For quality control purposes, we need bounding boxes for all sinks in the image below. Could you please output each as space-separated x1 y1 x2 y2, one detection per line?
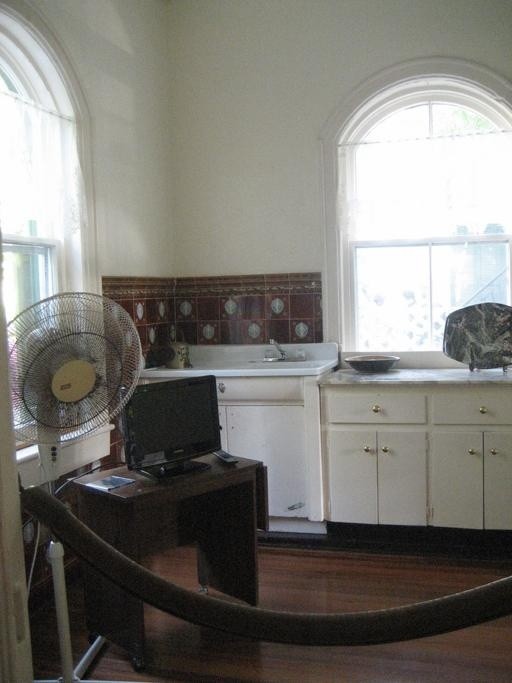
132 361 326 402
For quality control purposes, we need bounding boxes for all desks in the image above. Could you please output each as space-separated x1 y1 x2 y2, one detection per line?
64 452 269 667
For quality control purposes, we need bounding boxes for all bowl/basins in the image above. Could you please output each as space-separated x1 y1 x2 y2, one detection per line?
344 353 404 374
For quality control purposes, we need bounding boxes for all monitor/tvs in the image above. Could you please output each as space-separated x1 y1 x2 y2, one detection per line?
120 375 222 483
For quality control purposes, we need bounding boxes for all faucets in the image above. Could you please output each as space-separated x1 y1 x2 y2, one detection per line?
261 338 289 361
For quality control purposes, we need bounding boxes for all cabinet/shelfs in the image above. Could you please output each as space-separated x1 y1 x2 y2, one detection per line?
316 368 426 542
132 370 336 536
422 367 512 540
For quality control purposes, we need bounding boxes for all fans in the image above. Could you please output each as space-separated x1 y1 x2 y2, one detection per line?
7 292 145 683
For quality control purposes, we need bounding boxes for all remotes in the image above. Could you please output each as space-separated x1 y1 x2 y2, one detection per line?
213 450 239 466
